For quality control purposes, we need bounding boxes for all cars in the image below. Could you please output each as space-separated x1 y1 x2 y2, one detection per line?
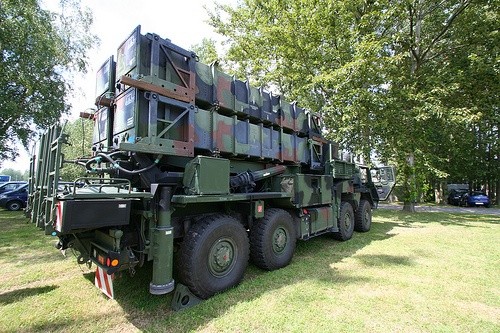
1 182 28 211
446 189 470 206
461 191 491 209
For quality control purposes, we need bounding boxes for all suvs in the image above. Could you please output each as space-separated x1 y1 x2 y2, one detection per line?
0 175 12 185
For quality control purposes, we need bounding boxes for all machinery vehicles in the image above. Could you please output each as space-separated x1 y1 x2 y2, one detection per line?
25 24 397 308
